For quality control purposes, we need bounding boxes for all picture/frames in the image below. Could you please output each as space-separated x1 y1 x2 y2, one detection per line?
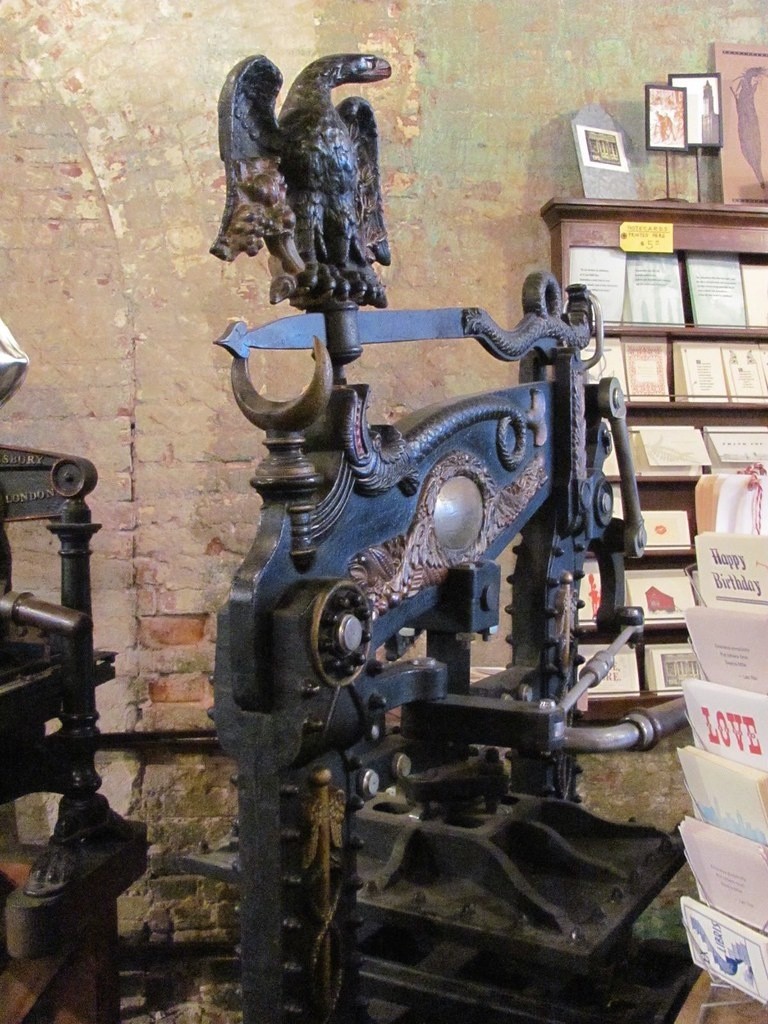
645 84 689 152
667 72 724 148
570 103 637 201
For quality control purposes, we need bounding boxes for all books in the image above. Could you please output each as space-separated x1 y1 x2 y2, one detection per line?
576 555 696 623
693 530 768 606
680 895 767 1005
677 745 768 847
609 476 768 550
579 335 768 405
681 676 767 774
600 416 768 476
684 605 767 696
573 642 710 698
566 244 767 331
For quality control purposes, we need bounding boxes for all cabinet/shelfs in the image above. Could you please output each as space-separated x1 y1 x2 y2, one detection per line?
540 197 768 728
0 445 150 1024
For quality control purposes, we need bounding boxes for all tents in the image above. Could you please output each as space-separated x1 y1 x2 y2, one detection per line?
678 816 768 936
